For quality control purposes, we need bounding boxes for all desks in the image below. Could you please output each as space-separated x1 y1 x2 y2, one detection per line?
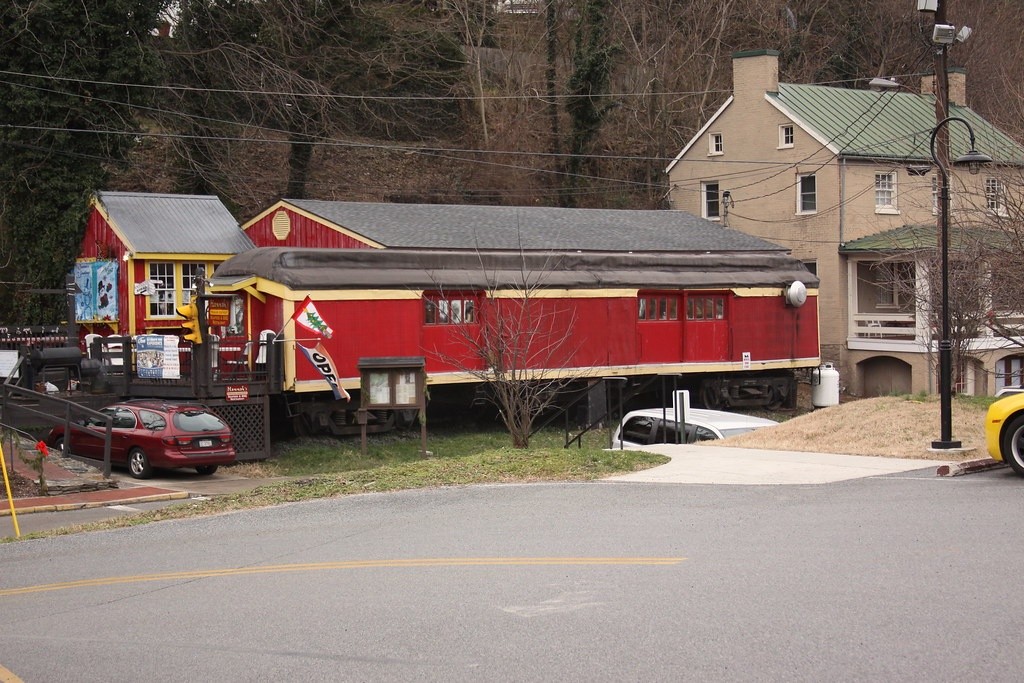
178 346 241 379
882 321 916 338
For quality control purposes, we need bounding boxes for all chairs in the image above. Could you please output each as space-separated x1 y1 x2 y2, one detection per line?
85 332 157 374
864 312 882 339
226 342 254 379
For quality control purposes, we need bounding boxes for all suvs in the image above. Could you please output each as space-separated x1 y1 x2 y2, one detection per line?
48 400 236 479
612 409 778 447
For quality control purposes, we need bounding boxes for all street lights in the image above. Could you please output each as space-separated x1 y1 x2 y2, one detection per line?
930 117 992 450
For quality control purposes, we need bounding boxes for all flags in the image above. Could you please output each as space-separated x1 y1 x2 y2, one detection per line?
297 343 352 404
291 296 333 339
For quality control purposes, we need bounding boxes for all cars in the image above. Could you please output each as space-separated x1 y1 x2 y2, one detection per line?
986 387 1024 476
